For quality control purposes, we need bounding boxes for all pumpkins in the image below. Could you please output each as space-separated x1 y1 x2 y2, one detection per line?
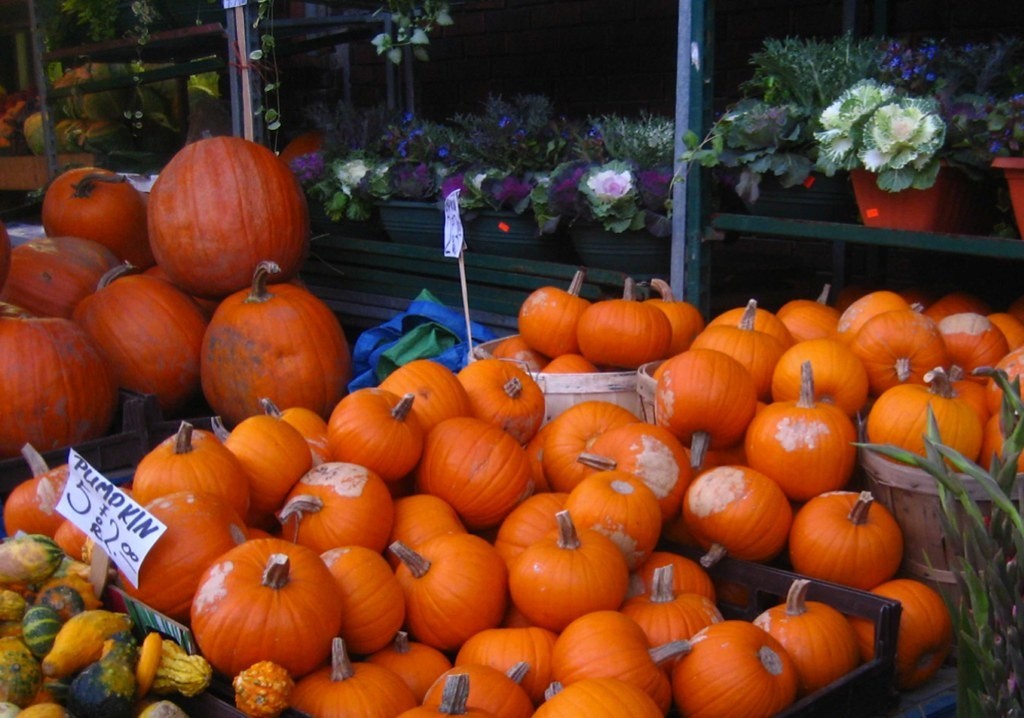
0 138 1024 718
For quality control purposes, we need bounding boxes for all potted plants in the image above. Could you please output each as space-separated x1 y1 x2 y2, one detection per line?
813 78 986 236
983 89 1024 241
664 97 861 223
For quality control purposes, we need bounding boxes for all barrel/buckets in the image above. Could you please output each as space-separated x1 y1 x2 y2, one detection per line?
467 332 638 425
857 413 1024 640
635 358 663 428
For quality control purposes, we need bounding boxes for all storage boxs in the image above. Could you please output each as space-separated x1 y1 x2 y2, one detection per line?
0 335 1024 718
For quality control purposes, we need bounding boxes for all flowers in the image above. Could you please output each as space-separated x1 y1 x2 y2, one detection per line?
441 165 558 235
290 143 397 222
530 148 673 247
387 160 448 201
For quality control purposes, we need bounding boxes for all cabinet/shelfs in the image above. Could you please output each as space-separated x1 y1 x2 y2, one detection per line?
683 0 1024 328
463 209 575 265
371 199 444 249
569 219 672 275
307 200 381 237
234 0 683 343
1 153 95 191
0 0 393 225
